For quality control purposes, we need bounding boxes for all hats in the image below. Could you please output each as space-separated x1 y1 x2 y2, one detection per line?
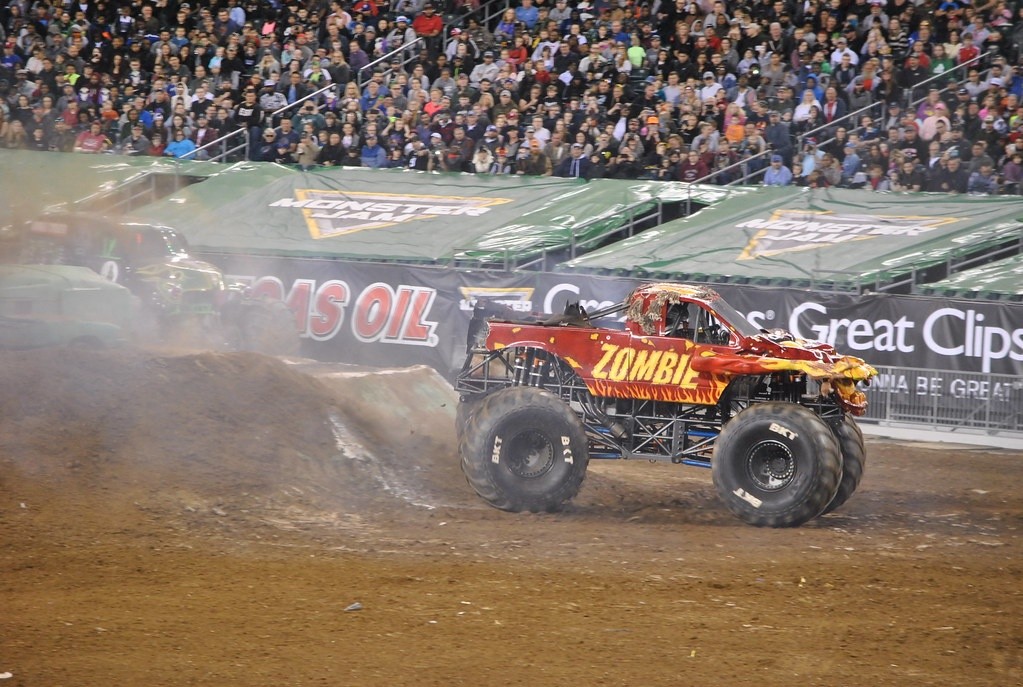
0 0 1023 196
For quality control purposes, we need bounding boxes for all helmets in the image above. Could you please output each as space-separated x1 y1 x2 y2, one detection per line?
666 303 689 336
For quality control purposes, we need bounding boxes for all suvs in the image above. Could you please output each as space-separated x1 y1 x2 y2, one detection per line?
22 207 248 347
455 284 882 531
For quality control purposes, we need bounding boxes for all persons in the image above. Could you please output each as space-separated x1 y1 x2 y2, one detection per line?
465 0 1023 196
666 305 727 345
0 0 485 173
